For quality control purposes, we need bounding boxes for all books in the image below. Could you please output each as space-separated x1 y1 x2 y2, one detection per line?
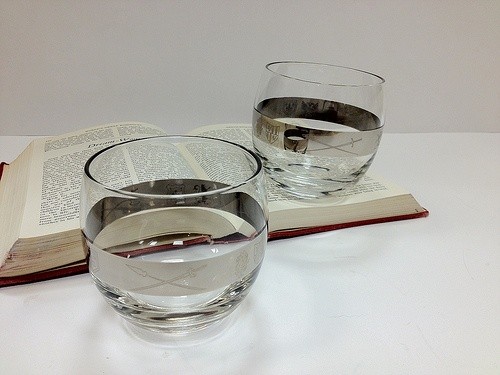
0 121 429 289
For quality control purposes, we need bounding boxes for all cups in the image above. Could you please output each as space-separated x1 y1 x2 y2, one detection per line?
252 61 387 205
79 134 268 334
